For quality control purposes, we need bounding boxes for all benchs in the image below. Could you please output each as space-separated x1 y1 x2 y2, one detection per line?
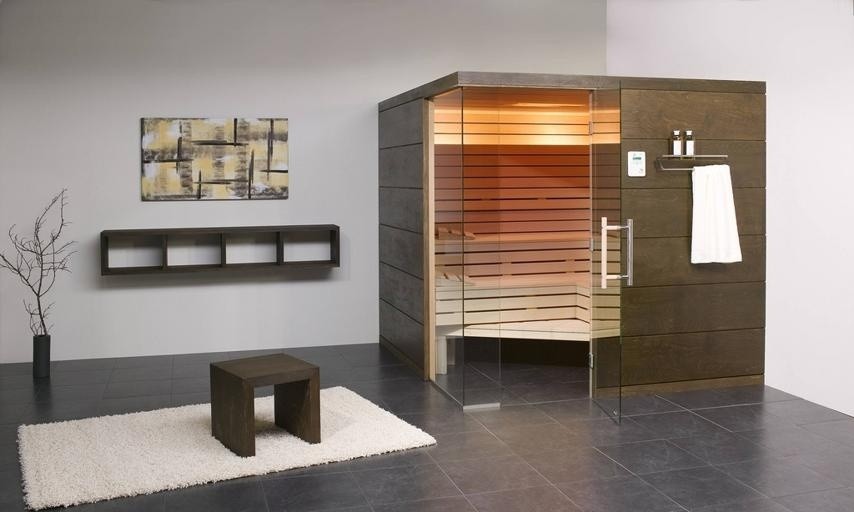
435 271 621 375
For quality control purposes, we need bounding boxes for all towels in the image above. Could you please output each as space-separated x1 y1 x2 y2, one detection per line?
689 164 741 266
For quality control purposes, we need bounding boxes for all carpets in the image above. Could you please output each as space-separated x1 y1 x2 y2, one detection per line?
10 381 436 511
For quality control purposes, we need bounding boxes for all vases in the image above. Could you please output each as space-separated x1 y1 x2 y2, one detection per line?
33 333 51 379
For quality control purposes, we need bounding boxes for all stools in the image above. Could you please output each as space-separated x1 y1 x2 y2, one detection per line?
204 349 321 458
